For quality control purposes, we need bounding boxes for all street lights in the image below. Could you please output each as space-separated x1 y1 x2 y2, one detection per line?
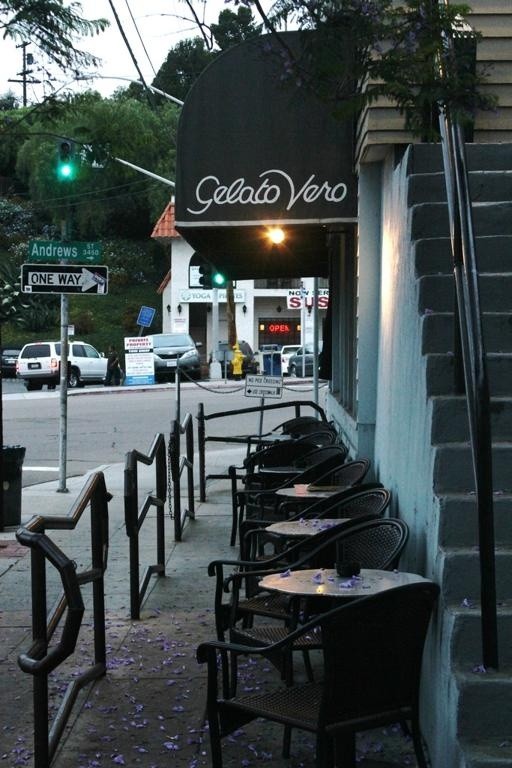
83 145 223 381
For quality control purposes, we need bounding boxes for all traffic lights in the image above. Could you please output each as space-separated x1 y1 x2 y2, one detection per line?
57 139 75 182
199 262 227 290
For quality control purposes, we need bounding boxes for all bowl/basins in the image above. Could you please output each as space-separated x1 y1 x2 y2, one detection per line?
289 431 301 439
270 430 283 436
335 561 362 577
294 484 309 494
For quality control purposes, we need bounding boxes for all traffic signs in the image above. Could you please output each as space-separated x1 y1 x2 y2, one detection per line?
21 239 110 298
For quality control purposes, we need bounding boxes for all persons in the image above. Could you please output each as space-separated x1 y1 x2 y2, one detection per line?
104 345 120 386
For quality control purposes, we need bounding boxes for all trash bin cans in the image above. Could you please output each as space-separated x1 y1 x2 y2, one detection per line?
261 344 281 376
0 445 26 527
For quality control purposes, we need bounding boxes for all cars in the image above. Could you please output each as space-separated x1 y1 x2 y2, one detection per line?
145 333 203 382
210 338 324 377
1 340 123 392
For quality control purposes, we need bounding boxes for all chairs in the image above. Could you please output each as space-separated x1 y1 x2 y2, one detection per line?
197 416 439 767
